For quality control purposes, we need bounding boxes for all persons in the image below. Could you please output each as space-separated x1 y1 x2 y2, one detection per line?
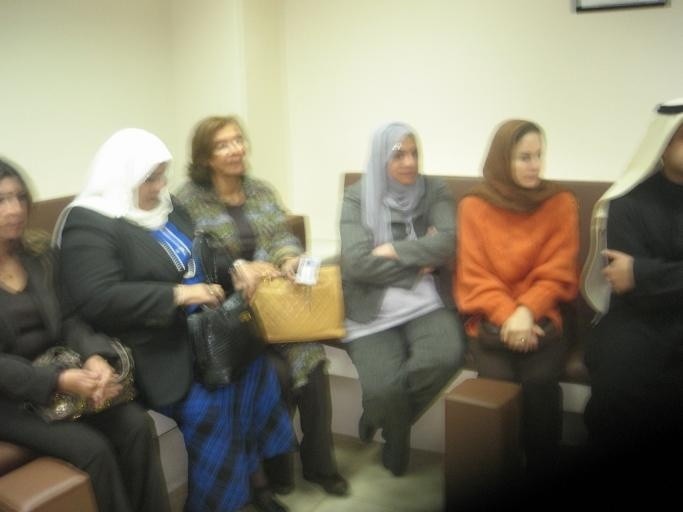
0 160 170 512
451 118 581 512
338 120 465 479
167 114 348 495
575 95 681 512
51 126 299 512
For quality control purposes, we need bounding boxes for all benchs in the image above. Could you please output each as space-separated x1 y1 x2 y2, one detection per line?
0 194 305 477
319 171 614 387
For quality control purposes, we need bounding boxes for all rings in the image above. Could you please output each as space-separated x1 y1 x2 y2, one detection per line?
518 337 526 343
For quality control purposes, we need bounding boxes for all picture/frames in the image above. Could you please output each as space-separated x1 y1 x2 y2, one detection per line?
576 0 667 10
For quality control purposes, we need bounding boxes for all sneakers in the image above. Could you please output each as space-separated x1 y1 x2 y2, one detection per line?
359 413 377 441
384 440 405 474
303 468 347 494
255 488 286 511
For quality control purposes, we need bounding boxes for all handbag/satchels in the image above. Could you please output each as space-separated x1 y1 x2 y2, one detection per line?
189 290 270 390
476 309 557 352
244 260 347 343
346 236 443 342
21 340 136 422
